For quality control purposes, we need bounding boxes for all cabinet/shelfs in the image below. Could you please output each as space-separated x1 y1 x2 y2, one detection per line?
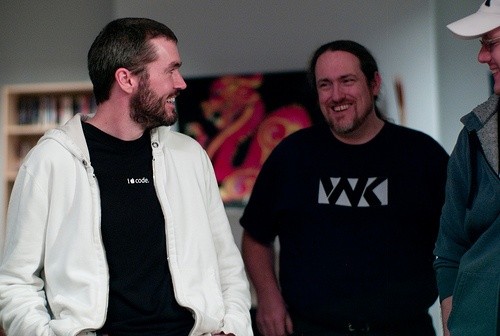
0 81 94 243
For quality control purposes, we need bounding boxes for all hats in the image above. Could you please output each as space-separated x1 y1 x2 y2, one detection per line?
446 0 499 39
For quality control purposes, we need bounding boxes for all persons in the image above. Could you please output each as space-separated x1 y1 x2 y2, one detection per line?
240 42 451 336
443 0 500 336
1 18 259 335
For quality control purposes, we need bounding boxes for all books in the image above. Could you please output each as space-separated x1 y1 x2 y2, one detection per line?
12 89 96 166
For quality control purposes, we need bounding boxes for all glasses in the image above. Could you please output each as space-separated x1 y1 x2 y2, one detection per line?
479 37 500 51
